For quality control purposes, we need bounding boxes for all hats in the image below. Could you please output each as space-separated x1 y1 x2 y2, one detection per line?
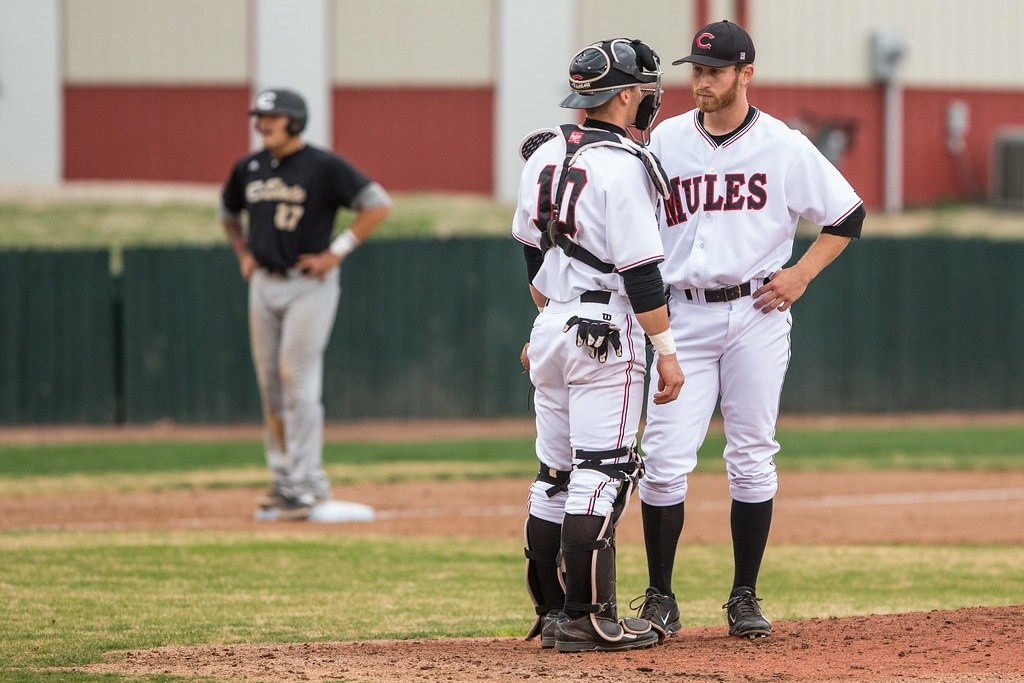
672 20 755 67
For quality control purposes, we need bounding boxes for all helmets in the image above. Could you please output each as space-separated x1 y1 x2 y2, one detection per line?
248 89 306 135
560 42 640 108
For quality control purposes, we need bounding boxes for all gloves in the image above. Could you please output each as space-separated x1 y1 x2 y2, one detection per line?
563 316 622 363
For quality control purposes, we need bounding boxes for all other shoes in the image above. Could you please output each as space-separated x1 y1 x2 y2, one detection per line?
256 486 319 519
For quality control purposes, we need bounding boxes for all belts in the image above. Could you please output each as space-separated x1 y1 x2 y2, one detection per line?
545 289 611 306
667 277 770 302
261 268 308 277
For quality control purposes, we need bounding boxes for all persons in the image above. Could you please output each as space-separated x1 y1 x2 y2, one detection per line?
639 18 866 636
510 38 684 653
221 88 391 520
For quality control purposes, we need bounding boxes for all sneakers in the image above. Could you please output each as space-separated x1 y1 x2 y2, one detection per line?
540 614 558 646
629 587 682 633
722 587 771 639
555 612 658 651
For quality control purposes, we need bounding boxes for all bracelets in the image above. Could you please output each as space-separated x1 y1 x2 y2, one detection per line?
648 327 676 355
